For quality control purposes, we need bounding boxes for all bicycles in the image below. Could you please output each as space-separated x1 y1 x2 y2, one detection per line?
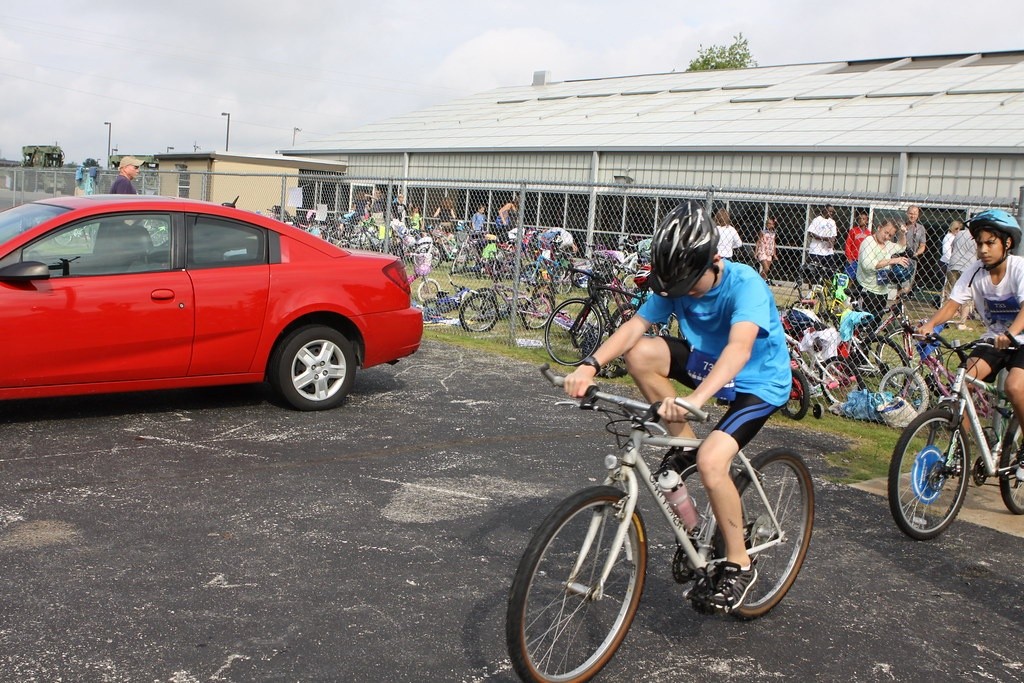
18 212 169 246
263 194 996 430
888 322 1024 542
504 363 817 683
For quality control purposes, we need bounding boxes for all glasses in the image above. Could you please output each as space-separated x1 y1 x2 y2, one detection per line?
132 165 139 170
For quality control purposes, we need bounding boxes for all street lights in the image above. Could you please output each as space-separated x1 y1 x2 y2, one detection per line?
221 112 230 151
104 121 112 169
166 146 175 153
112 147 119 155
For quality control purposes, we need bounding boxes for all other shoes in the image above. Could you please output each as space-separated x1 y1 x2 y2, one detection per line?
1015 457 1024 481
954 447 963 466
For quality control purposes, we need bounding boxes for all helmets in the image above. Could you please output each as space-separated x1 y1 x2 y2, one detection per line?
969 209 1022 251
633 266 653 288
649 200 718 297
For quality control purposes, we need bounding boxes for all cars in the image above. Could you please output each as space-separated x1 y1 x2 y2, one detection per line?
1 192 426 412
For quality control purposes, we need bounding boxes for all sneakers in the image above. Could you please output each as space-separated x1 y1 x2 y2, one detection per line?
653 444 700 483
707 561 758 609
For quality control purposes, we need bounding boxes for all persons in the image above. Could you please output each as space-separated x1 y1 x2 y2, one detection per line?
354 190 512 239
93 156 145 253
806 203 978 376
563 201 792 611
912 208 1024 486
714 208 742 258
754 217 778 279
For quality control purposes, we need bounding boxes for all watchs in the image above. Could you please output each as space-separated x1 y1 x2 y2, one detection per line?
584 356 601 377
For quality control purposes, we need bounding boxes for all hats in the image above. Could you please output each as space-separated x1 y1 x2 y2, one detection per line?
118 156 144 169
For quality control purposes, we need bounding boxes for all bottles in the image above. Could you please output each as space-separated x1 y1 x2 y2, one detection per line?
657 470 700 532
